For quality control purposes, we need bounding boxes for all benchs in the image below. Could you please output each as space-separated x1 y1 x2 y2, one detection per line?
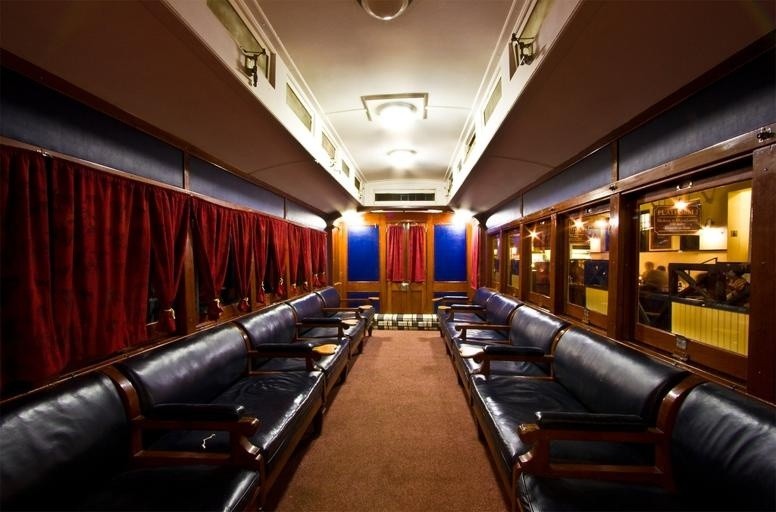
316 286 379 343
106 323 324 499
453 304 570 406
462 324 685 504
430 287 496 336
286 292 370 371
236 304 348 410
442 294 523 358
511 377 776 512
0 365 265 512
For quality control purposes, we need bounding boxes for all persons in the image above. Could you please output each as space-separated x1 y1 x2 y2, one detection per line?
643 261 752 307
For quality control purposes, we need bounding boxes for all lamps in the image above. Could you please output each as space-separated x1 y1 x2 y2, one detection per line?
359 93 429 123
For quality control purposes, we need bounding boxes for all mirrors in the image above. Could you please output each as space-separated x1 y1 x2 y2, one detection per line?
490 234 500 284
508 228 520 289
528 219 552 299
638 178 753 356
567 211 610 315
